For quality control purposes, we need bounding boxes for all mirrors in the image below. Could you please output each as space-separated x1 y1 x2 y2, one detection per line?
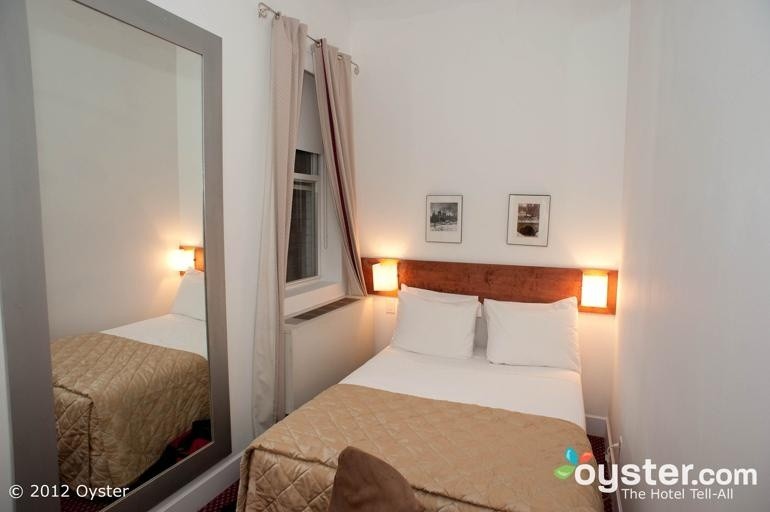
1 0 233 512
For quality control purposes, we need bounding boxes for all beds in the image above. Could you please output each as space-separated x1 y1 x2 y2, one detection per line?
242 346 608 512
47 313 211 496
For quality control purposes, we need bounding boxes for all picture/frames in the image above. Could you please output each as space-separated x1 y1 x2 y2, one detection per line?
425 194 463 243
508 192 550 247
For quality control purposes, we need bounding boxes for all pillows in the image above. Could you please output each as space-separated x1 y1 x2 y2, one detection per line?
171 268 206 319
391 284 583 372
